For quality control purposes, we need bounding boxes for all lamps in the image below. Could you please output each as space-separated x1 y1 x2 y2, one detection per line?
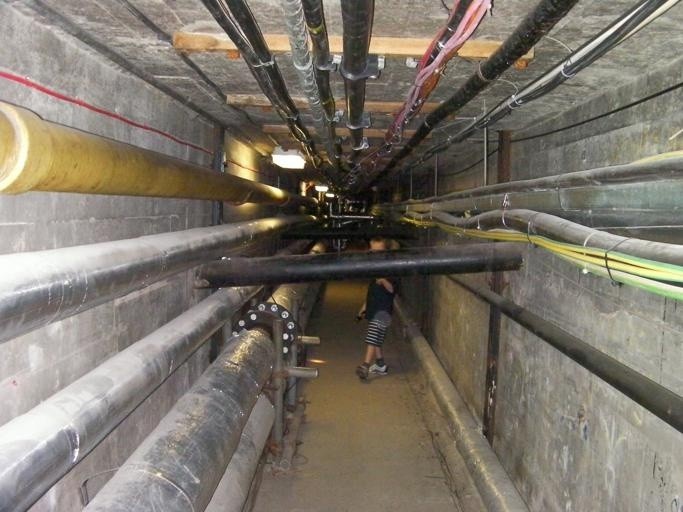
270 141 308 170
314 183 330 192
324 191 335 199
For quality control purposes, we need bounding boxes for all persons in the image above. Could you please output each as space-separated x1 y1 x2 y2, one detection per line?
356 276 397 383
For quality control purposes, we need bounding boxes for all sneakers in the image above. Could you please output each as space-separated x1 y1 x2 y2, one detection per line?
369 365 388 375
357 362 369 382
